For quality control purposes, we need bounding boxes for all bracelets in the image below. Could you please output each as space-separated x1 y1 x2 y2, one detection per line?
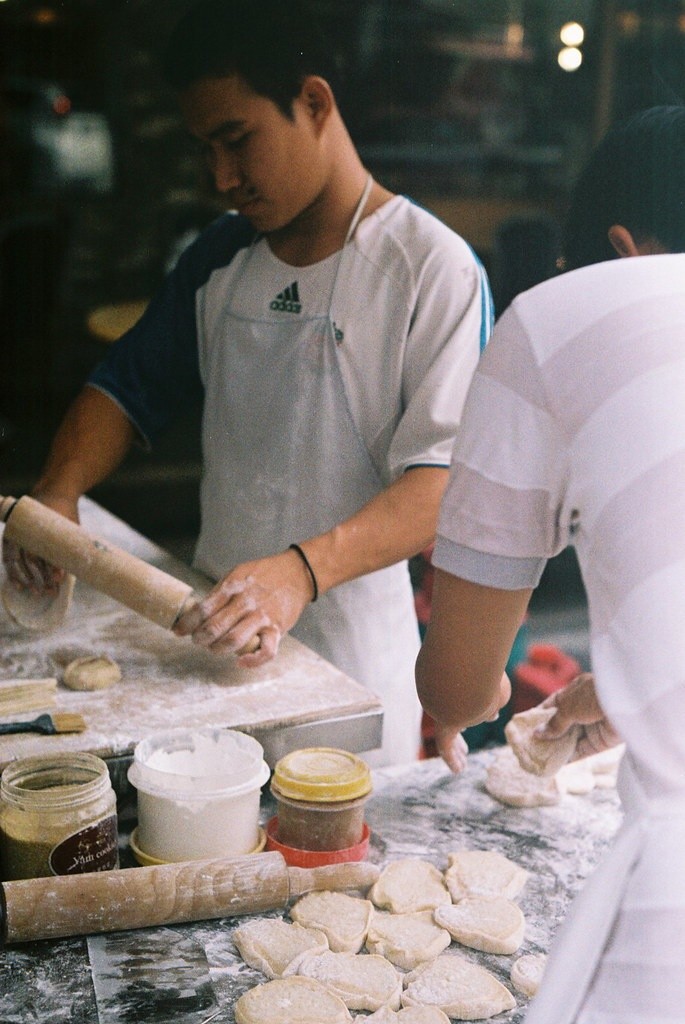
289 544 319 602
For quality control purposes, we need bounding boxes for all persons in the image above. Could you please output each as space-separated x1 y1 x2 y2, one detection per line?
415 104 685 1024
0 0 494 771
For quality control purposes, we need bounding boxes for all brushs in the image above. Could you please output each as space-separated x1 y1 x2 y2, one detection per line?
0 713 86 735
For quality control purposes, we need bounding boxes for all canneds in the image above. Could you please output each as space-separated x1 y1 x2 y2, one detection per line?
0 751 120 882
